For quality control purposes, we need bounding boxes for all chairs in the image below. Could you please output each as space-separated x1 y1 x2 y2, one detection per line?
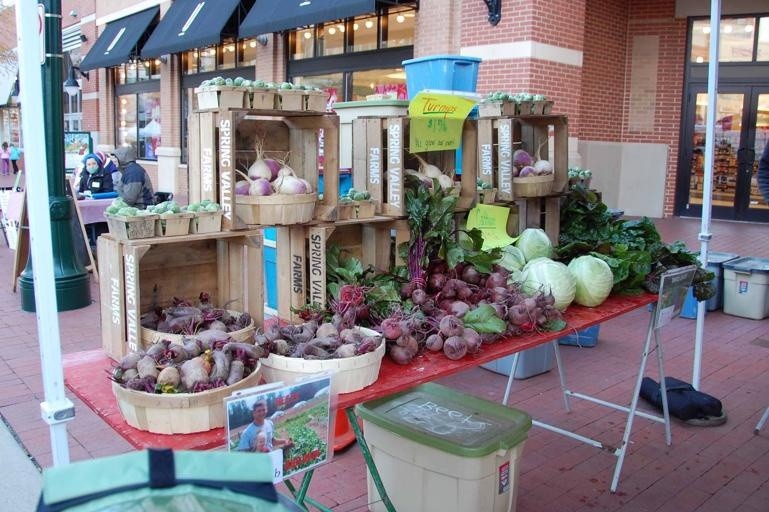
153 191 175 206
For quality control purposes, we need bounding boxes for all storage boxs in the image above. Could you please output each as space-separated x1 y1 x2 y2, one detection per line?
355 382 531 512
478 338 557 380
404 57 481 98
676 266 708 319
695 251 740 312
560 324 600 349
724 256 769 320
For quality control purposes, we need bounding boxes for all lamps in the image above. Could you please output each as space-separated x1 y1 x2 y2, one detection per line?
64 66 89 97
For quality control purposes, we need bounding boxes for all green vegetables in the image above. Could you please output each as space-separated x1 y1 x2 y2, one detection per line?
553 184 716 303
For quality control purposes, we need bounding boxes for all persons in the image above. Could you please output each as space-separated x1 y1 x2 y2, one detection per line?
8 142 21 176
78 154 113 194
237 400 294 455
110 146 156 211
0 141 11 176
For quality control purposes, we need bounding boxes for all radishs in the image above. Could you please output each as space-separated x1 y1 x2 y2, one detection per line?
234 137 312 195
512 144 552 178
404 150 452 190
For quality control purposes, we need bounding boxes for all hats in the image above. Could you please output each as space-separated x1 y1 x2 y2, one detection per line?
82 154 103 165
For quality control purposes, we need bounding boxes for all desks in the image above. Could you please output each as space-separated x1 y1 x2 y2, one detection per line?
77 194 117 236
62 256 695 494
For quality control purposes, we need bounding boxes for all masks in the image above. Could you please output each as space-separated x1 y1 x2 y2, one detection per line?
86 164 100 175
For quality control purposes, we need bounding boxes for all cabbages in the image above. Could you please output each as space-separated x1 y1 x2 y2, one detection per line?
497 226 614 312
